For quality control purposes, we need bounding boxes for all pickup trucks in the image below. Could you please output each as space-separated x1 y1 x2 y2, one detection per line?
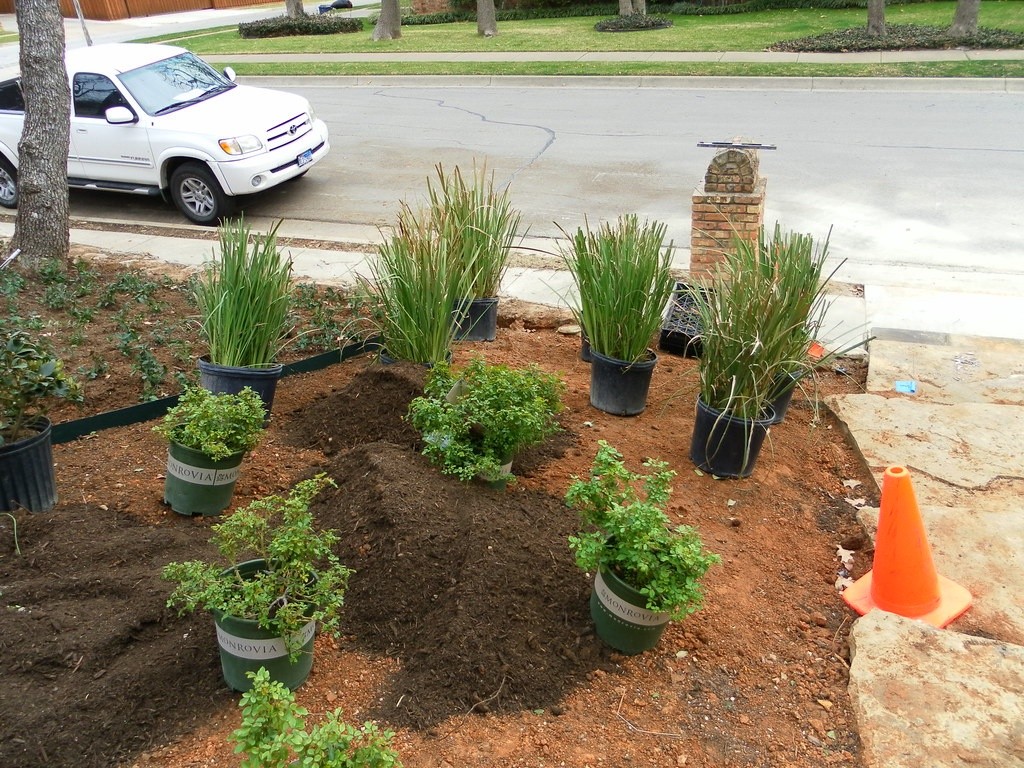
0 43 332 227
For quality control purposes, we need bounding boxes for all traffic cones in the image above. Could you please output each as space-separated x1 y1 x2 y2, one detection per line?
844 467 976 634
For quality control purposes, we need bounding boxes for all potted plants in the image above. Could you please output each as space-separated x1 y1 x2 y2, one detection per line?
403 350 571 491
539 186 876 478
162 472 357 694
0 331 83 514
188 211 320 429
564 440 722 656
339 154 532 371
152 386 271 519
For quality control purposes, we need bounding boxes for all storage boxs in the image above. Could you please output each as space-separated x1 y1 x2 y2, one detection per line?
661 281 718 358
